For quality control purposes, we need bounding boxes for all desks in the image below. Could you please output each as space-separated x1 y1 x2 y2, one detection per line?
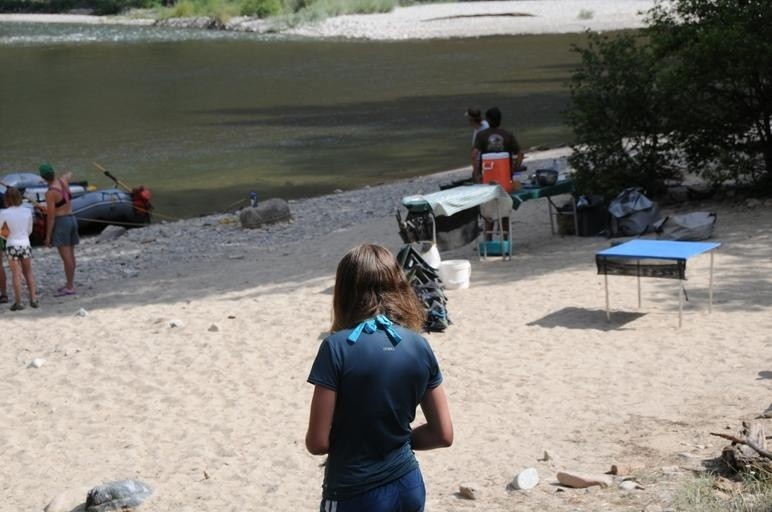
480 179 581 263
594 238 723 329
401 184 508 265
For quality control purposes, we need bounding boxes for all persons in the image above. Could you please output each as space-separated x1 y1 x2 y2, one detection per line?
39 164 79 296
0 188 38 309
464 106 490 169
304 246 454 512
471 106 523 241
0 192 9 303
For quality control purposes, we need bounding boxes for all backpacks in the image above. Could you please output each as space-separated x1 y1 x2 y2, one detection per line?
131 185 153 216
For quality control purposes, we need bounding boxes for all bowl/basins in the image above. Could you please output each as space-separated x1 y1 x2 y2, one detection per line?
536 167 559 186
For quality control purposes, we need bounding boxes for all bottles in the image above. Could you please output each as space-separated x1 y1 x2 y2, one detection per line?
530 172 536 183
250 191 258 207
512 171 521 191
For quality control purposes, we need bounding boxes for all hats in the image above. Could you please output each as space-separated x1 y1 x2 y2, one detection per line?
463 111 482 121
38 163 55 180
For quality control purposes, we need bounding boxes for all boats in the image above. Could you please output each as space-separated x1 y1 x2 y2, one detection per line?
0 172 154 246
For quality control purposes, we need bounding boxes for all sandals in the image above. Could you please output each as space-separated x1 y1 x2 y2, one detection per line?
54 287 75 296
29 298 38 308
9 302 25 311
0 295 8 303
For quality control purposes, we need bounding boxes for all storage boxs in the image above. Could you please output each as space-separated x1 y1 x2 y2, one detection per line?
557 203 609 237
478 240 511 256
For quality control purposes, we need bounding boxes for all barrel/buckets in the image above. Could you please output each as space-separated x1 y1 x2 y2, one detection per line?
439 258 471 289
